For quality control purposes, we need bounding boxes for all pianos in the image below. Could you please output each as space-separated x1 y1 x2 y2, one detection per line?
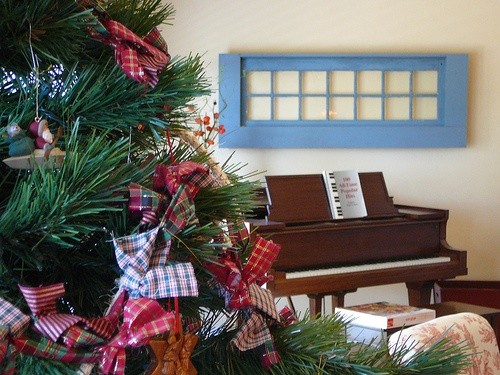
244 173 468 324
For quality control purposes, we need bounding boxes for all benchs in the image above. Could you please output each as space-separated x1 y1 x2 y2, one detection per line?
326 300 500 348
